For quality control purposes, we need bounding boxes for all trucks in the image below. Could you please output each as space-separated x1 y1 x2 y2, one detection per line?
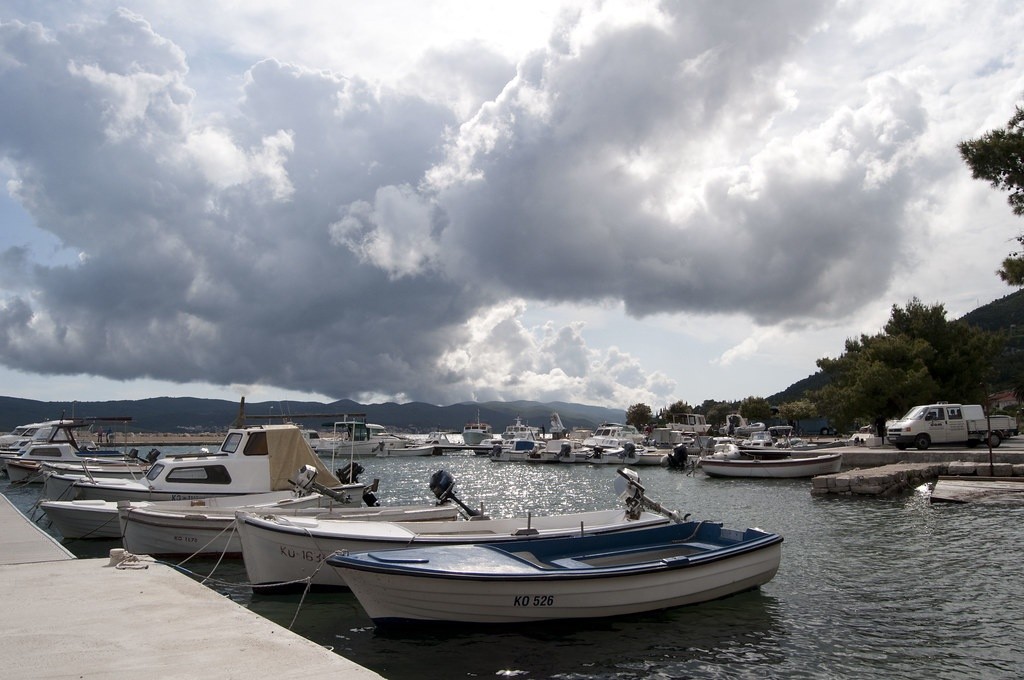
886 401 1018 450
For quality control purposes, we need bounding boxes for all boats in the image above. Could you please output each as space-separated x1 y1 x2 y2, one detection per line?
37 482 322 538
115 470 490 560
324 520 784 630
42 424 365 508
701 454 843 478
233 468 687 595
0 419 161 482
299 410 818 466
